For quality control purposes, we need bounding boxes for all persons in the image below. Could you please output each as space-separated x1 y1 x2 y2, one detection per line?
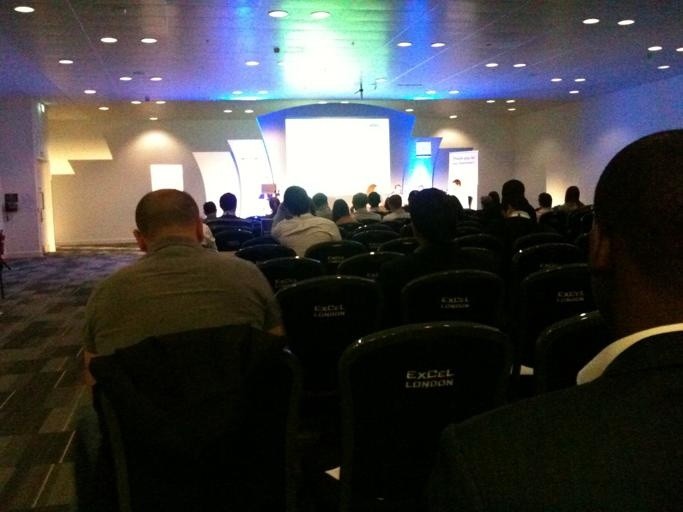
451 180 469 209
203 193 237 221
434 128 683 510
481 180 584 222
269 183 458 257
72 189 282 441
